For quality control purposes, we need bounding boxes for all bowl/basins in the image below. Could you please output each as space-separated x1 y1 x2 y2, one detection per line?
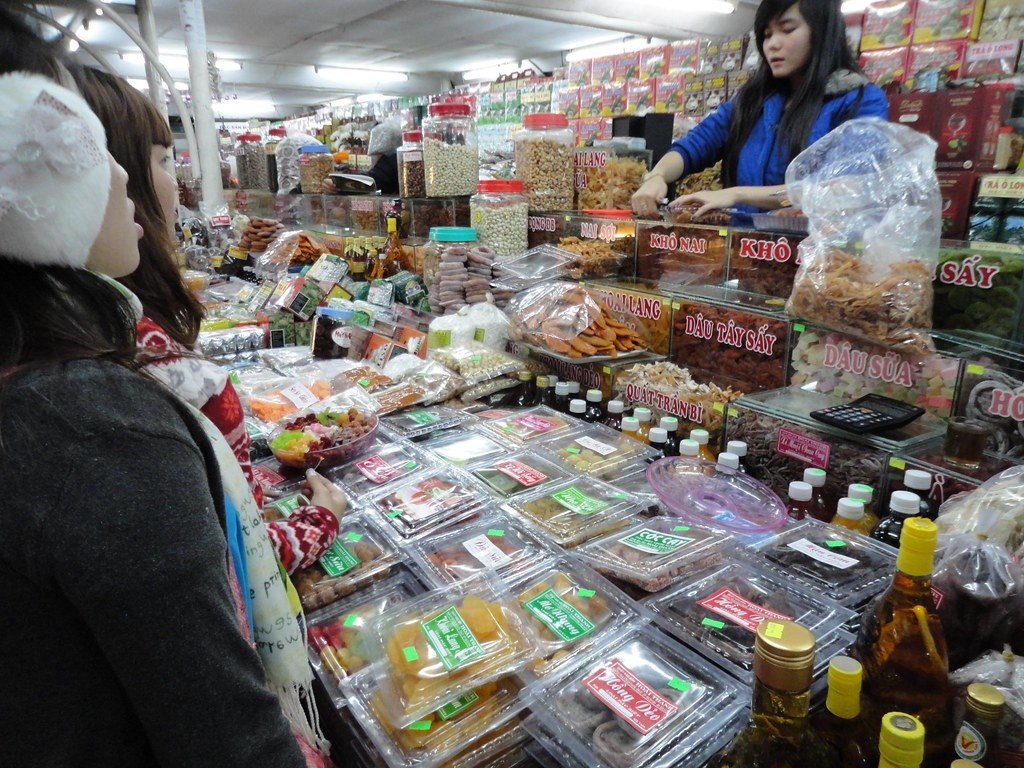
267 409 379 469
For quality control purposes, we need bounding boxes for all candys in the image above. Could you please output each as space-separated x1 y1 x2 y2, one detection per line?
792 332 960 416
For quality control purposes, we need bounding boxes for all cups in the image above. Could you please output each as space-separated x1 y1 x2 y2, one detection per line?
943 415 991 470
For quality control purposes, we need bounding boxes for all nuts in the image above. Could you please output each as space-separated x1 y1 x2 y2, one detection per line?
515 139 575 211
400 159 427 197
470 201 529 258
933 250 1024 347
422 137 479 197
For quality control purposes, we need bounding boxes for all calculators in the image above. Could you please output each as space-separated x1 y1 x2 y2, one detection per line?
810 393 924 434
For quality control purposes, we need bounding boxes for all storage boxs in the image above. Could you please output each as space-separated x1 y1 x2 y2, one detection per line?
559 1 981 122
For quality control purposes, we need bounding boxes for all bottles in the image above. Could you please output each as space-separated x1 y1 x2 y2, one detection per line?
992 126 1012 170
516 370 1006 767
236 103 574 285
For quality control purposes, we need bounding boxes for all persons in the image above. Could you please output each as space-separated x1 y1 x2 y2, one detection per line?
0 1 333 768
630 0 890 218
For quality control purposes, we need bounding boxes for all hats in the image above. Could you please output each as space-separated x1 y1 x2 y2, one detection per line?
0 71 111 268
